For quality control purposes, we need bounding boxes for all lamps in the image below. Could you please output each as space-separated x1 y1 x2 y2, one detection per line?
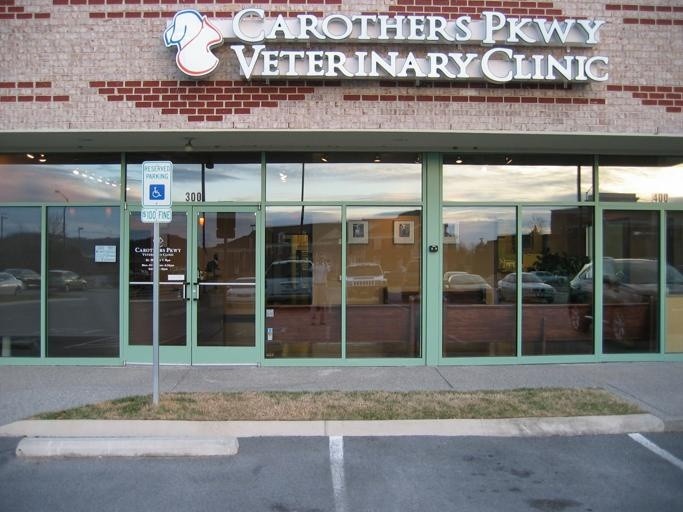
455 153 464 165
319 153 331 165
503 156 513 163
374 153 381 163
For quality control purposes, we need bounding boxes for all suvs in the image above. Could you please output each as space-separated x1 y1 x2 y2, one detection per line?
566 256 683 350
265 257 317 307
338 261 393 307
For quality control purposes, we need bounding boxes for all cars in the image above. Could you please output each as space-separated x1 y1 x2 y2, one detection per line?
0 265 111 298
397 255 571 305
226 275 256 308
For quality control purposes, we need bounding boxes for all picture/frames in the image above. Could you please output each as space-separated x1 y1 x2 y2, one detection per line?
345 218 371 247
443 220 461 247
391 219 417 245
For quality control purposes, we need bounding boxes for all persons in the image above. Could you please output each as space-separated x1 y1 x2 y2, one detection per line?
307 252 332 326
205 252 222 272
